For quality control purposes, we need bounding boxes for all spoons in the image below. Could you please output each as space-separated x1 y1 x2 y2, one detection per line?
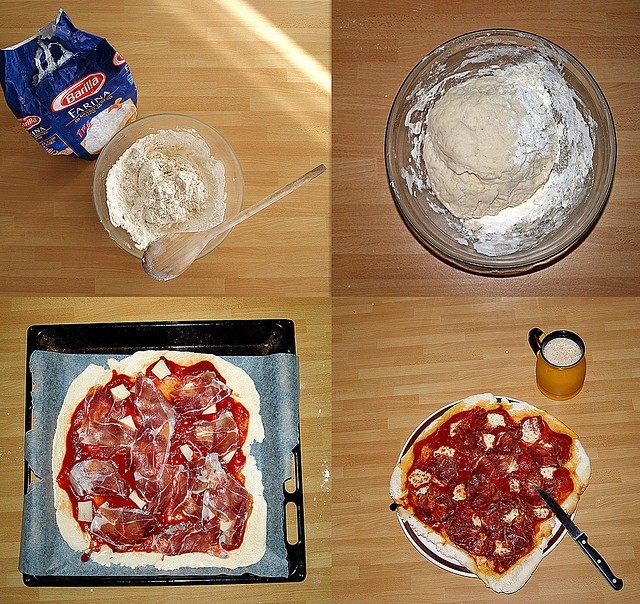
141 163 329 284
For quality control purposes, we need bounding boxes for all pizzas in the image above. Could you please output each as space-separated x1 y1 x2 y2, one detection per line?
389 391 589 594
50 347 268 570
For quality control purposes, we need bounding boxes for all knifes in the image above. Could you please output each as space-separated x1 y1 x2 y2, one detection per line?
536 487 626 591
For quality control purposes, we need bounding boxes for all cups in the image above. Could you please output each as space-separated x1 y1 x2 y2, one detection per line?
524 325 587 402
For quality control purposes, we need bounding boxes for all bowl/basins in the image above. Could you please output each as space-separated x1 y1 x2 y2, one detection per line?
92 114 244 267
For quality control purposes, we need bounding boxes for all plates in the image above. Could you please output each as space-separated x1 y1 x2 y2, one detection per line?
22 316 310 588
382 29 619 279
390 395 577 576
